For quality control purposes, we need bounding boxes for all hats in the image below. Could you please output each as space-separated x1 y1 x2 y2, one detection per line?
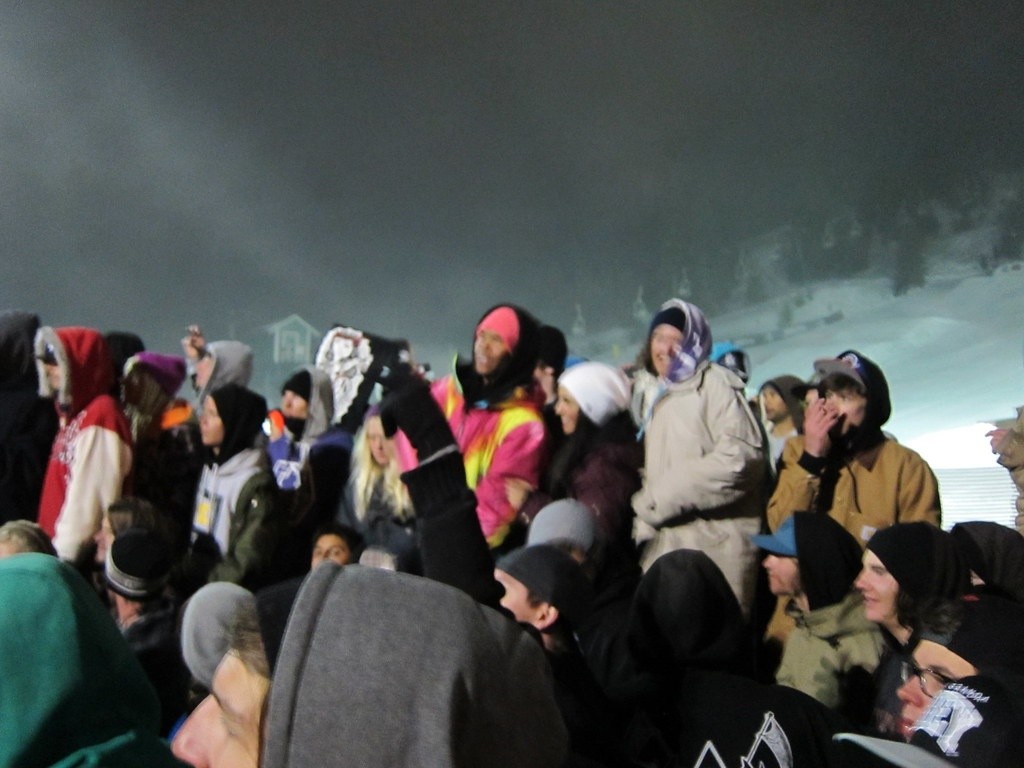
495 543 582 625
558 360 633 429
475 306 521 355
104 528 169 602
37 343 59 366
281 370 312 402
813 351 866 387
647 307 690 339
527 498 595 554
751 516 799 558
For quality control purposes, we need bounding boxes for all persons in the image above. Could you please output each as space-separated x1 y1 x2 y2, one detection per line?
0 297 1024 768
170 563 568 768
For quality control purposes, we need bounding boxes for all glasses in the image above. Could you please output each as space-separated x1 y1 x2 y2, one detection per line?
898 655 953 701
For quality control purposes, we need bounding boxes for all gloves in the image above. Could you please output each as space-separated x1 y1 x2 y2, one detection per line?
381 367 459 463
